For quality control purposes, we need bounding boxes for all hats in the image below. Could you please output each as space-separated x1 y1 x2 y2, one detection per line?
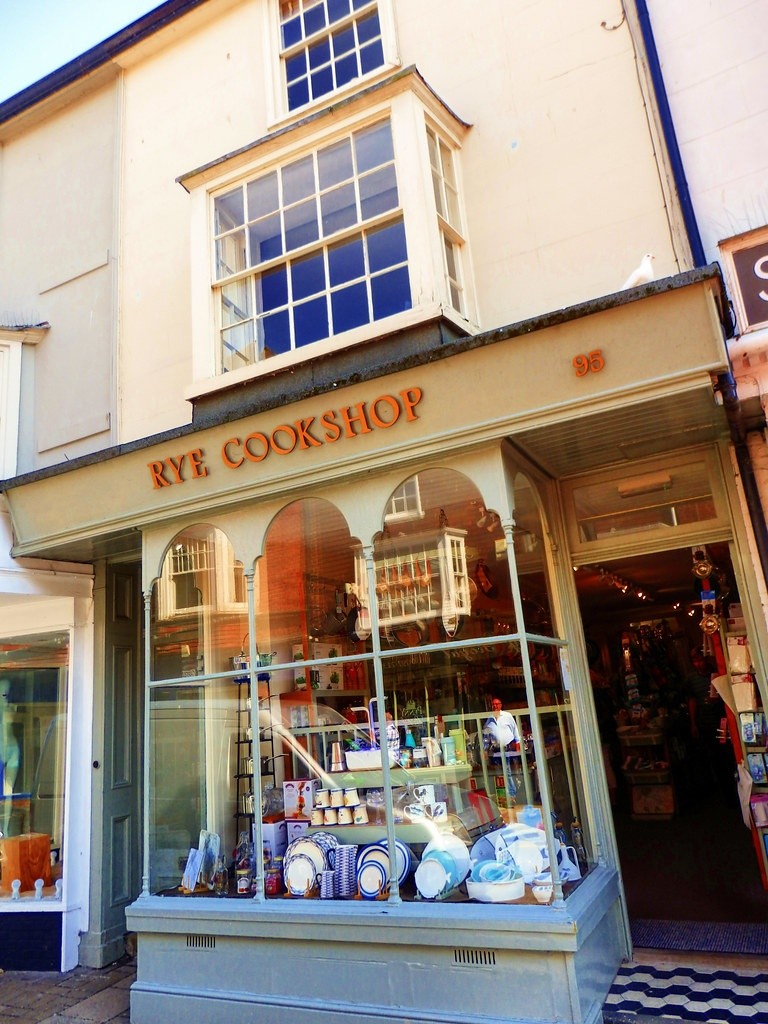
384 712 394 720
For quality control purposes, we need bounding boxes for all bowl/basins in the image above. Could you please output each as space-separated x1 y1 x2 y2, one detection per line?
465 877 525 903
534 871 570 886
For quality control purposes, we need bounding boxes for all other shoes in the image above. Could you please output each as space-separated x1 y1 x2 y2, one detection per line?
516 778 521 791
509 796 517 808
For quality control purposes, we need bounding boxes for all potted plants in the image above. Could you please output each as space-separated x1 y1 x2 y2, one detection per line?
293 643 344 691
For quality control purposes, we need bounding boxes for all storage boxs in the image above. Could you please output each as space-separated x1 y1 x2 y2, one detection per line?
619 733 664 746
623 771 673 785
252 778 322 856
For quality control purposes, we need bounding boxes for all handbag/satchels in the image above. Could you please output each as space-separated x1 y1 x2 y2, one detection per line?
735 764 754 830
474 732 490 751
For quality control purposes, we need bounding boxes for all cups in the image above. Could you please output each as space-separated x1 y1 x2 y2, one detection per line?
310 785 370 825
402 784 448 823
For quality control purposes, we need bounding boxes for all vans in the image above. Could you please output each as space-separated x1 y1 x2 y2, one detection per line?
2 699 507 877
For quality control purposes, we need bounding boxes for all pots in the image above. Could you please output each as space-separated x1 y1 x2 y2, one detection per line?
241 693 289 815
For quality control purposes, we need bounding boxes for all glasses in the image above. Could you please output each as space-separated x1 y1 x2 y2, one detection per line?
492 703 502 706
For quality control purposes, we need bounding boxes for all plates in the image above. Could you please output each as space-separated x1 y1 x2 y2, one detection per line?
279 832 472 900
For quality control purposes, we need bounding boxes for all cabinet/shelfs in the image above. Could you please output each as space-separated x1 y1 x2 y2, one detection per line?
289 764 475 845
348 529 470 627
717 591 768 891
233 677 276 846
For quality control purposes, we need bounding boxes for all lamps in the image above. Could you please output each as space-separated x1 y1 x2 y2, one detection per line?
487 513 498 533
477 507 487 528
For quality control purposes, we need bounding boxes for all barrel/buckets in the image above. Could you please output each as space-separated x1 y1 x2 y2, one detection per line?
229 633 278 680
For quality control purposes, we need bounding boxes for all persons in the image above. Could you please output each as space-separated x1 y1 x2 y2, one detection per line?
386 712 401 761
687 646 738 811
483 698 521 797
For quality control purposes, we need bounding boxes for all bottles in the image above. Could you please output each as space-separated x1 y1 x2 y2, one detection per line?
233 839 284 893
398 727 469 768
572 822 586 862
214 856 228 895
554 822 570 861
